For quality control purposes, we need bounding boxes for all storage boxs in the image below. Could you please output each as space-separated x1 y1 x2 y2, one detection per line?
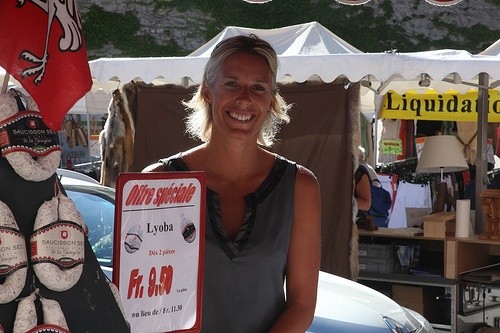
358 259 394 273
358 241 394 260
423 212 455 238
391 285 435 322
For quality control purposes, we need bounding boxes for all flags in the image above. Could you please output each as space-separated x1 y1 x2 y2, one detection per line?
0 0 93 132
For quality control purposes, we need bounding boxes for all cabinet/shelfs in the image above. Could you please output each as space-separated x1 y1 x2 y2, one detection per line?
356 227 500 333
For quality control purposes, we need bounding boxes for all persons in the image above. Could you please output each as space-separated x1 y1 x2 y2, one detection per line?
487 137 496 170
358 146 381 188
139 35 321 333
353 164 371 226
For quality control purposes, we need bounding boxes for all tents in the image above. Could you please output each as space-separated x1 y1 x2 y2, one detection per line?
84 19 500 235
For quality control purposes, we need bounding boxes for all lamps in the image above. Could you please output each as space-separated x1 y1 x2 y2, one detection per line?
416 135 470 182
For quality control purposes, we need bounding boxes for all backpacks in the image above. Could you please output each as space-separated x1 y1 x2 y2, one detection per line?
368 178 392 227
